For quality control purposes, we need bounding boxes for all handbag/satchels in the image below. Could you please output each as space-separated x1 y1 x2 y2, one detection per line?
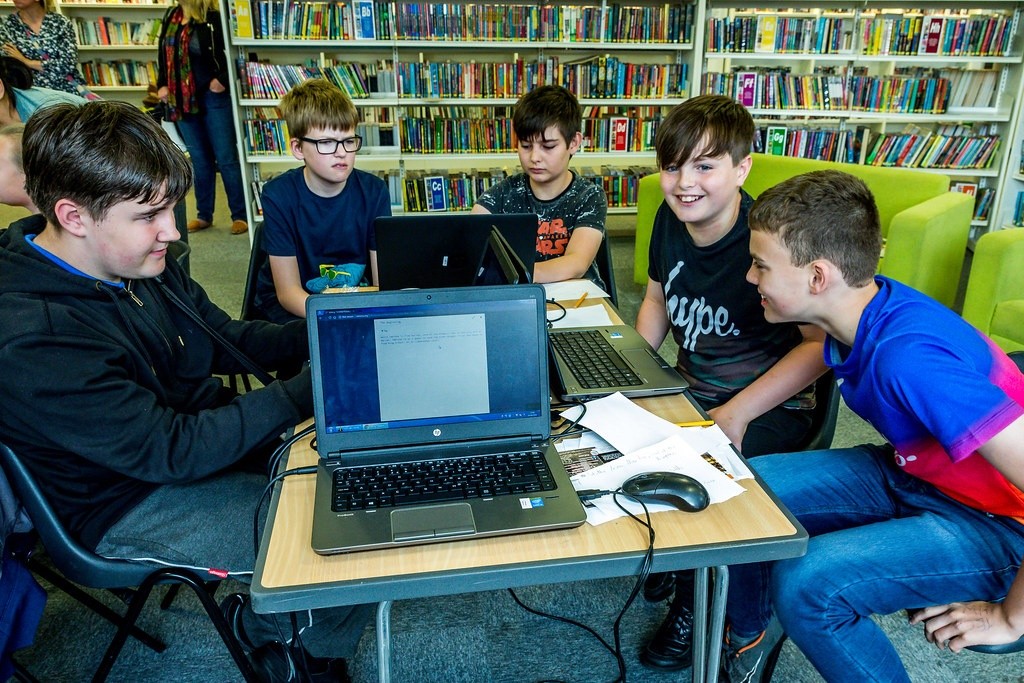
84 91 103 101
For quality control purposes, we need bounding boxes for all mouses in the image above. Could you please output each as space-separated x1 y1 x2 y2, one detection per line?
621 471 711 513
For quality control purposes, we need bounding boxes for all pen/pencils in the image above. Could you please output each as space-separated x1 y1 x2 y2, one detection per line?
674 420 714 427
574 292 588 308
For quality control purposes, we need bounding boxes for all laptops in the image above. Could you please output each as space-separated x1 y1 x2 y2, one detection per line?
305 213 689 555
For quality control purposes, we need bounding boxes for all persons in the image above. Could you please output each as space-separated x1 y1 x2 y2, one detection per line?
640 93 823 675
0 2 611 683
707 171 1024 682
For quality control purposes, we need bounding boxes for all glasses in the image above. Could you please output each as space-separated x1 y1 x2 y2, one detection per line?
298 134 362 155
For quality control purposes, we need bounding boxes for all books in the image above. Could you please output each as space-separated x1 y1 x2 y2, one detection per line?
49 1 1024 211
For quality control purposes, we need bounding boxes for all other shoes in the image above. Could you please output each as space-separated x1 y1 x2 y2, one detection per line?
231 220 248 233
219 593 256 653
186 218 211 232
250 640 355 683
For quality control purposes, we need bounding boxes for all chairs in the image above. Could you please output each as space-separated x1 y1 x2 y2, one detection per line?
0 444 255 682
595 232 619 309
238 222 263 321
760 351 1023 683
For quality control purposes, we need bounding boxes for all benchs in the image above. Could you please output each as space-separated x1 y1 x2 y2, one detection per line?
962 227 1024 357
632 151 975 309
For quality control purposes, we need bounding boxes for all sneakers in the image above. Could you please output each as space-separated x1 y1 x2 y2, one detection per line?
717 609 785 683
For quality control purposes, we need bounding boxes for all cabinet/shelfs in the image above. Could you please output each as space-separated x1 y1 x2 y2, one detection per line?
0 0 1024 253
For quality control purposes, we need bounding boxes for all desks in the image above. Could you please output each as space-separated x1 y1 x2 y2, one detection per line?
250 287 809 683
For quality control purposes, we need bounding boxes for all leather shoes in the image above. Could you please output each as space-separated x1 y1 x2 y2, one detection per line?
643 571 676 602
640 598 711 672
